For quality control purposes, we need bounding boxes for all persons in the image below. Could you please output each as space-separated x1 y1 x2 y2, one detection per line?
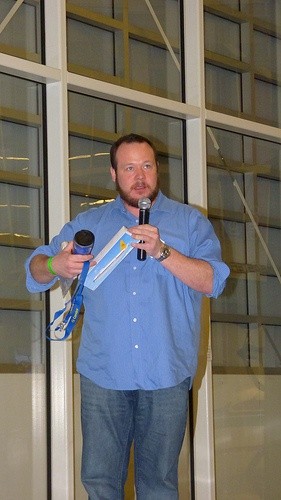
24 133 230 500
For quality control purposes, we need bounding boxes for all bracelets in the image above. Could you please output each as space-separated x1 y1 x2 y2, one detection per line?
48 256 59 276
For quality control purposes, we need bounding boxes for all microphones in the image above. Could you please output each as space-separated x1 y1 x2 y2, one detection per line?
138 197 151 261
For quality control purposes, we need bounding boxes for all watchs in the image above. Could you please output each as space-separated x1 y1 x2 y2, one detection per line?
156 243 171 262
